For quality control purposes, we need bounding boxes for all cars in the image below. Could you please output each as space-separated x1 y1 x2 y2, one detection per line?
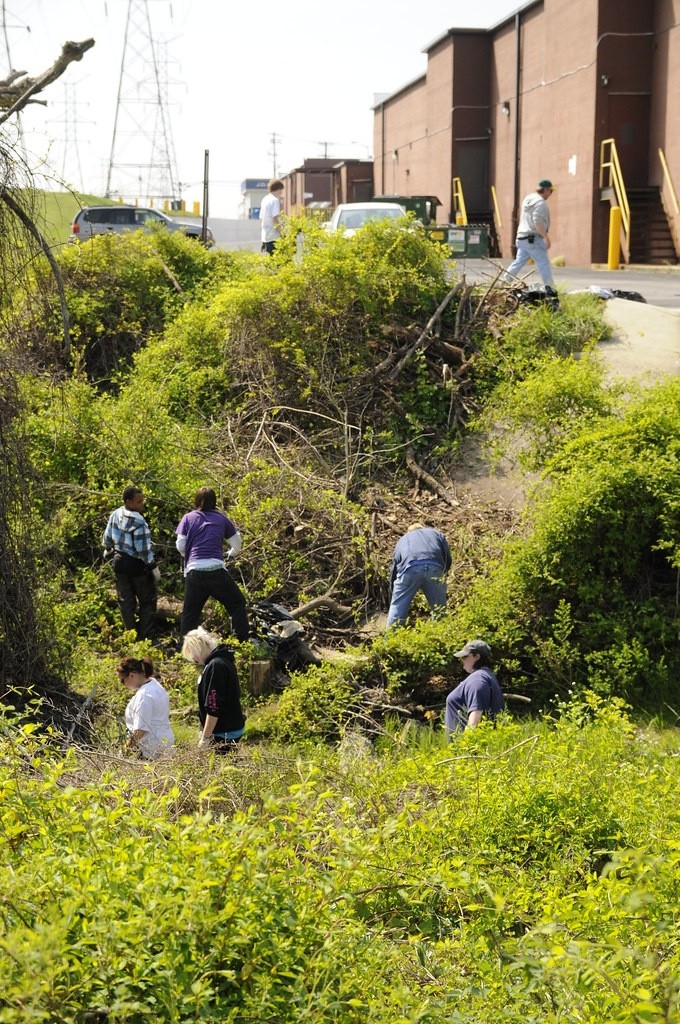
320 201 421 246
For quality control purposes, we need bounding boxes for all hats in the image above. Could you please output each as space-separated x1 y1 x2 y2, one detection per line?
453 640 491 658
539 180 557 190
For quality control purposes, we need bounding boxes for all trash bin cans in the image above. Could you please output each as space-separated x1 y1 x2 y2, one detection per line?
424 223 489 260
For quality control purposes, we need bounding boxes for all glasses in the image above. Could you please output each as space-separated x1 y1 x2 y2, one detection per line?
194 658 199 664
121 675 126 685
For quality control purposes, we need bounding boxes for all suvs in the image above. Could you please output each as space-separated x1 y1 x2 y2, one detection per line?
68 206 214 251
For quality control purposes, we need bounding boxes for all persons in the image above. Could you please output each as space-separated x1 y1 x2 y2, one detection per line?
385 523 452 638
180 625 247 755
497 179 560 312
116 657 176 762
443 639 506 746
101 485 162 643
258 179 286 257
175 485 250 654
